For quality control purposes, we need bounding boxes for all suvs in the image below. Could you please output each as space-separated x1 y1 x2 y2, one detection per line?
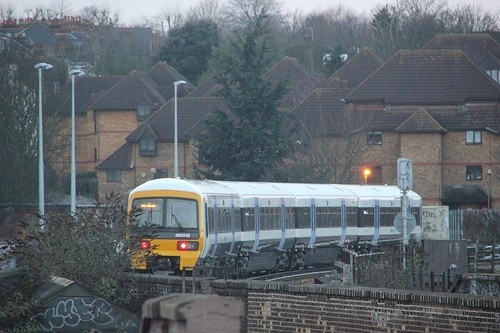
441 183 496 210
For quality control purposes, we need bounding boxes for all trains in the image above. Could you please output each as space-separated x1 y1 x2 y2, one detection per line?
125 177 423 277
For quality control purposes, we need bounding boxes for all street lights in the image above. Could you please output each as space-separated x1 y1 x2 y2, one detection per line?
34 63 54 229
174 80 186 178
69 69 85 217
